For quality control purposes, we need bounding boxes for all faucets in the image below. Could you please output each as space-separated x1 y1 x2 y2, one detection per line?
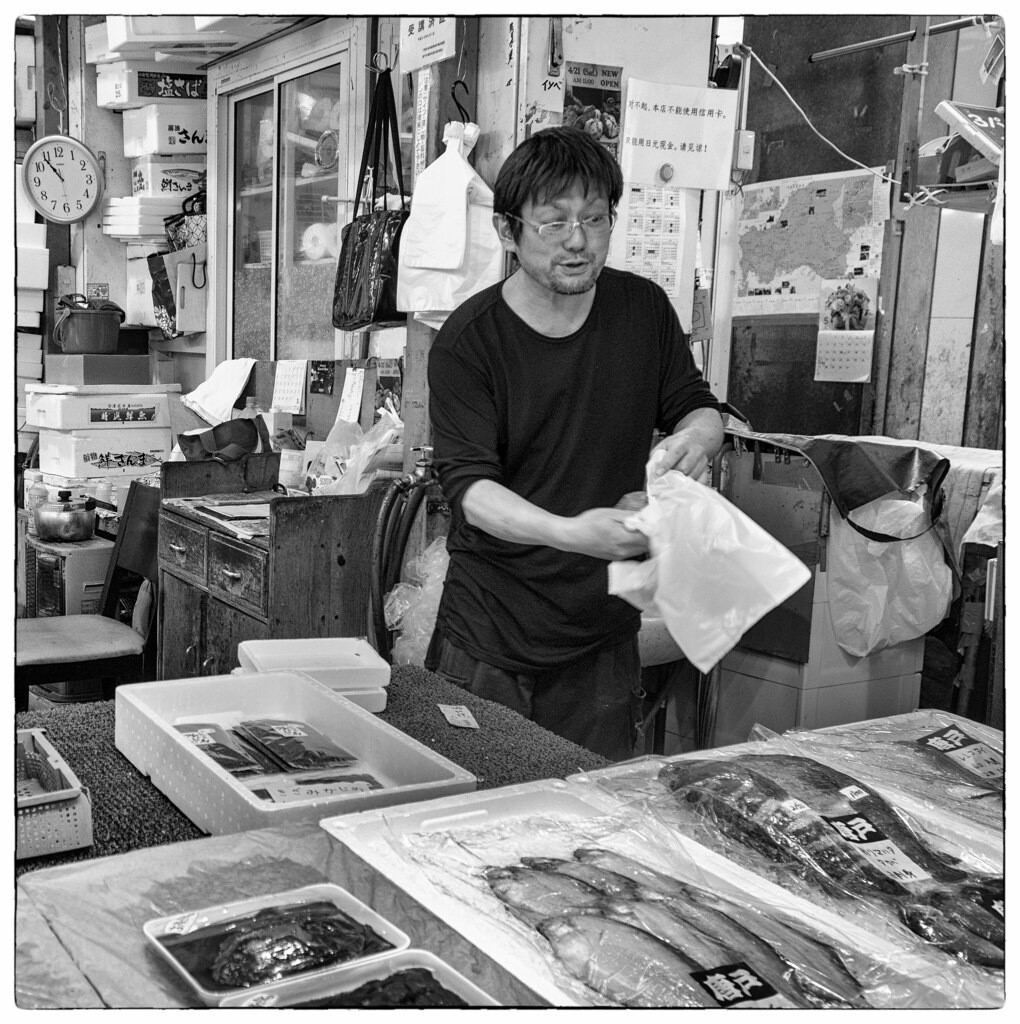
406 443 439 489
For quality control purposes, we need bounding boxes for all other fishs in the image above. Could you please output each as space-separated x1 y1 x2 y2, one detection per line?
486 755 1003 1008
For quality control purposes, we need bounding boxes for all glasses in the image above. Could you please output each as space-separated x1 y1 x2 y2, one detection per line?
505 207 618 242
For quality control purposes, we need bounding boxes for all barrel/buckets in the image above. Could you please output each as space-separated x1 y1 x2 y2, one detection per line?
53 308 122 354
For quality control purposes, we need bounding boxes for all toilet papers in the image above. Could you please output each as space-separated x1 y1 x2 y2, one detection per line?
307 96 341 132
302 222 337 260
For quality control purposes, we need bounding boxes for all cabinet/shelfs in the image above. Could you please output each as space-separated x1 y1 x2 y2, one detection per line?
236 71 340 270
155 453 388 680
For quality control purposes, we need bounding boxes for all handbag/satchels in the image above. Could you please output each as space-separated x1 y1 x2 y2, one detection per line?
146 190 207 339
332 210 411 333
176 415 272 460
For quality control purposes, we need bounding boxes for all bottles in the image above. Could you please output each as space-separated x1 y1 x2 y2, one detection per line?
28 473 49 536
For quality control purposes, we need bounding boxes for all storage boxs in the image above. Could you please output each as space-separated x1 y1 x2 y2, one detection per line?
16 15 307 506
16 726 98 861
115 670 476 838
230 637 391 713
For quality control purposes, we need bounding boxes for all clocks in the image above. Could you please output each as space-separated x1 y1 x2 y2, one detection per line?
20 133 104 225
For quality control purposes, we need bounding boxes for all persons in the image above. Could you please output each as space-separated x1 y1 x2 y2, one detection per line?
420 126 725 762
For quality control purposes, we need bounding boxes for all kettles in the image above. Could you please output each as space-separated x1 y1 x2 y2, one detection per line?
33 490 97 542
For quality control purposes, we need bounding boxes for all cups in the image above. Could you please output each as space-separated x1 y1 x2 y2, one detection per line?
299 127 339 169
